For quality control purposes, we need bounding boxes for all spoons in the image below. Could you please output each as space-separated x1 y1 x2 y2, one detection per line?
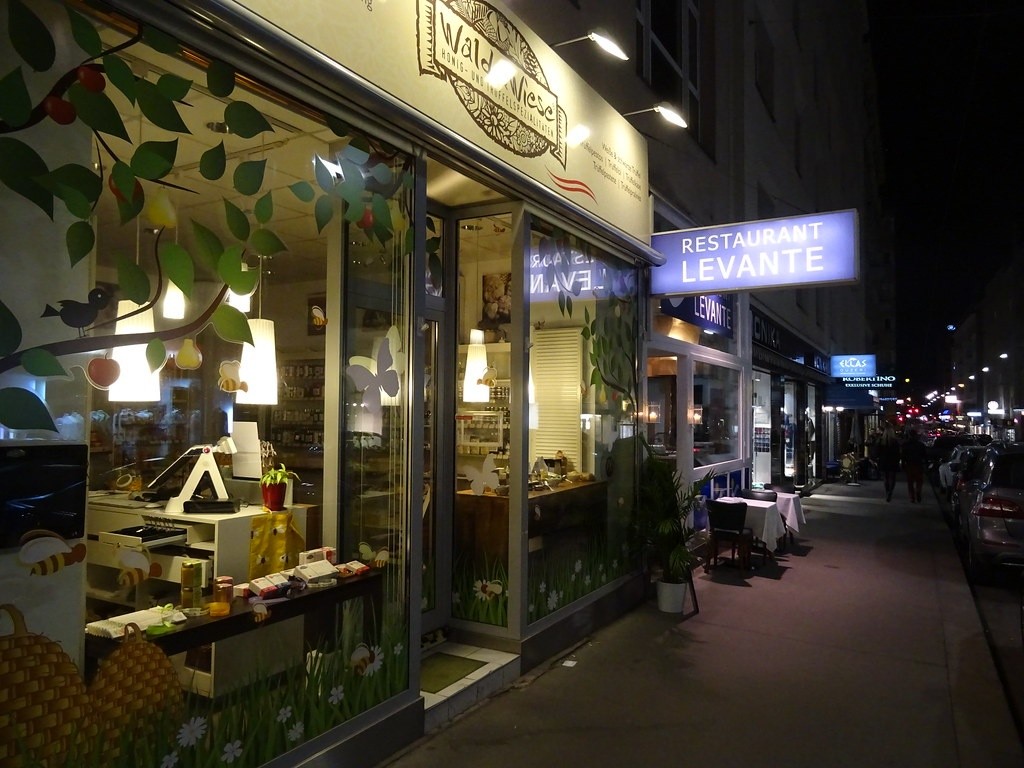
544 481 554 492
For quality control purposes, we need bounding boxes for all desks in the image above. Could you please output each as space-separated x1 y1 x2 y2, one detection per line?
84 571 384 686
706 496 786 567
456 478 609 615
751 488 807 546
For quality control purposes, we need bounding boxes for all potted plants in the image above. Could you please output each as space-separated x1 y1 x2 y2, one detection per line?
638 447 718 614
259 463 302 511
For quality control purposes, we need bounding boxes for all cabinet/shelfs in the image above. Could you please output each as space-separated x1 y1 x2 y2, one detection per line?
93 368 191 480
87 490 292 612
269 342 512 460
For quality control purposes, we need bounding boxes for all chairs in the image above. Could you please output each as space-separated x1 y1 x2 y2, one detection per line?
743 488 778 502
704 498 753 575
763 483 796 494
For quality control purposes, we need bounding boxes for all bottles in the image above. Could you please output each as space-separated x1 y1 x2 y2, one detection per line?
274 408 324 422
54 412 84 440
113 408 154 441
485 407 510 429
281 361 324 376
458 387 463 401
539 456 548 479
172 407 185 422
490 387 509 402
126 443 136 459
271 426 323 444
284 381 321 398
457 433 510 455
92 410 105 442
181 562 193 608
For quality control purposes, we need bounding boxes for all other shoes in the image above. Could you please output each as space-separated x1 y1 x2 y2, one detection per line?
916 491 921 503
910 497 915 503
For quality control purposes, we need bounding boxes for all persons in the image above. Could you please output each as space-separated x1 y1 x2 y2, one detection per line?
901 430 927 502
871 425 901 502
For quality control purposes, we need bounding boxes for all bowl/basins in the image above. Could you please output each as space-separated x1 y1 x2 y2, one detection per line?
567 475 581 481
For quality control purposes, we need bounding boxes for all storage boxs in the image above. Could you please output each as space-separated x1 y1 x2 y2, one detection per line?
233 546 370 598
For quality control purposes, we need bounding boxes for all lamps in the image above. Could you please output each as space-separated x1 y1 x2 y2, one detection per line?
163 172 186 320
548 25 630 62
228 262 251 313
369 335 402 407
621 101 688 128
461 217 490 403
107 108 161 403
235 131 278 406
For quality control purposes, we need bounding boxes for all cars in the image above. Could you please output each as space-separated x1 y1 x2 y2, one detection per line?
930 434 993 494
950 441 1024 576
